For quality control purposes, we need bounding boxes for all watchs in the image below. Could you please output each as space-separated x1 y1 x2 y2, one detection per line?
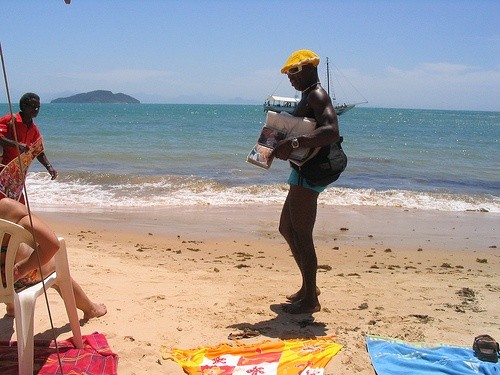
292 139 299 149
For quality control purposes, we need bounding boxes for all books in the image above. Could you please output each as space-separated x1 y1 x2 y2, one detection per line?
246 127 287 169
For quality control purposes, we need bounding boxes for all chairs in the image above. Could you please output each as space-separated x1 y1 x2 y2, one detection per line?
0 218 83 375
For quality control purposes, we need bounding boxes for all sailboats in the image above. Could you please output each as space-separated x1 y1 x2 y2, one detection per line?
263 56 368 116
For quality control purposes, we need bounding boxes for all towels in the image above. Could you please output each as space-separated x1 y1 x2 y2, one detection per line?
159 334 345 375
0 331 119 375
365 335 500 375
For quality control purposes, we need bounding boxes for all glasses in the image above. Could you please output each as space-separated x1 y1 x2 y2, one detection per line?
287 64 308 75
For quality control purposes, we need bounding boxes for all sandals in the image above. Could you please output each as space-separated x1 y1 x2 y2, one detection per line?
474 334 498 361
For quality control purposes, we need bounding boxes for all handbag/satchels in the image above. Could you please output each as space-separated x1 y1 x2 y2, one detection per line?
290 136 347 180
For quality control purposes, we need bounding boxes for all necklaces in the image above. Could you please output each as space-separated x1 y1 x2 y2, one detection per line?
303 82 321 93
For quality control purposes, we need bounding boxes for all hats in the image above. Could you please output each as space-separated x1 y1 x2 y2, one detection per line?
281 50 320 74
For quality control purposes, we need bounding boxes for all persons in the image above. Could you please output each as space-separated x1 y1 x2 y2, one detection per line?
0 198 107 320
273 50 340 314
0 92 57 204
